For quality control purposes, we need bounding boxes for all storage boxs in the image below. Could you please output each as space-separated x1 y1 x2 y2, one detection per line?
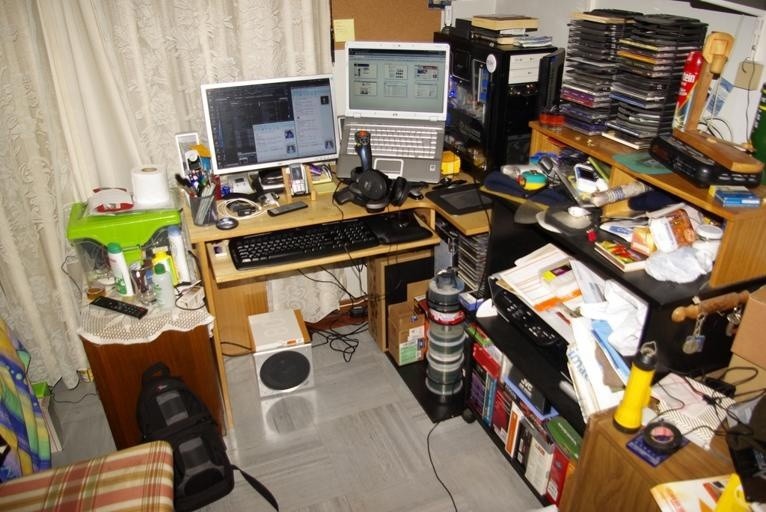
69 188 190 291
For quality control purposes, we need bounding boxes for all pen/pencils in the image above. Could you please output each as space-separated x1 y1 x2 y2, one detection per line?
175 167 219 227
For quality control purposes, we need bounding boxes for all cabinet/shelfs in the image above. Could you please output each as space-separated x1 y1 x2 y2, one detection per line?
82 293 226 454
422 122 766 511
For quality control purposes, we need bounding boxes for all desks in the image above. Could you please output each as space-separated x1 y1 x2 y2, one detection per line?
178 156 493 432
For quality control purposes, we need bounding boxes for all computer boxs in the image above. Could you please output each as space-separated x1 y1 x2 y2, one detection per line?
434 32 558 183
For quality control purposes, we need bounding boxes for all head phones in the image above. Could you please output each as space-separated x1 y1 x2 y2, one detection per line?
351 166 408 206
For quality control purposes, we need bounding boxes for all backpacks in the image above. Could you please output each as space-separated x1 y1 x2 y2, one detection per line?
134 361 238 511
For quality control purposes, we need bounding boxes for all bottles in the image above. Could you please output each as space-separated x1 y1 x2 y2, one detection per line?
107 242 133 297
612 340 658 435
671 50 705 134
168 226 191 283
152 264 176 309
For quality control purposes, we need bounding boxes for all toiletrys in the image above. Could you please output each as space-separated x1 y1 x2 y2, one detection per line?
152 263 176 312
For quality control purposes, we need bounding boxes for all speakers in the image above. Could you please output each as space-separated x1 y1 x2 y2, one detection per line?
175 132 200 174
338 116 345 140
247 309 315 398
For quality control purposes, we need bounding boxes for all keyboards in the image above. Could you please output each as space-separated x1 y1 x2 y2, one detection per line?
228 216 380 271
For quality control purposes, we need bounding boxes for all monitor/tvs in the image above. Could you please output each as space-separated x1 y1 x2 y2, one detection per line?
200 73 341 191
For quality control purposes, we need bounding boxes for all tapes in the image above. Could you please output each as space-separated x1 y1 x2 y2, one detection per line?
424 276 466 404
643 421 683 455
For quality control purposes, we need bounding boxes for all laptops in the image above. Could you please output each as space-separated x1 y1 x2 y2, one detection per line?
336 41 450 184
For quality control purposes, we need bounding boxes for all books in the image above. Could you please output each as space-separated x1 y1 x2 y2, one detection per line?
472 33 516 45
471 14 537 31
567 346 625 416
475 27 526 37
593 238 646 272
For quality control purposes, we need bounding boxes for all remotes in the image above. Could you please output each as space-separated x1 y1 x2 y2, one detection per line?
267 201 308 216
90 295 147 320
